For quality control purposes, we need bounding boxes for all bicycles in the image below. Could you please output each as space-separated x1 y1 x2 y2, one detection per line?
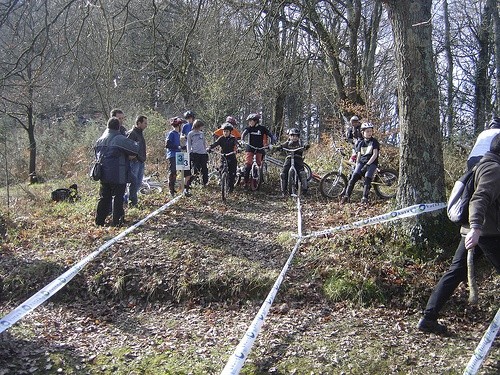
241 142 272 191
206 148 241 199
278 144 312 196
319 147 399 200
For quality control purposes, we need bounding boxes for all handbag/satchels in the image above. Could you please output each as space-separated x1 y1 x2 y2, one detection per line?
89 162 102 181
51 188 76 203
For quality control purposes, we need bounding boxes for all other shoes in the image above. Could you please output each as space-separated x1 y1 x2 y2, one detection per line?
361 198 368 203
418 318 447 335
340 196 350 204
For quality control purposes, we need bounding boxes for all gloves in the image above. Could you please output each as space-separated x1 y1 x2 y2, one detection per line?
304 143 310 150
361 164 370 173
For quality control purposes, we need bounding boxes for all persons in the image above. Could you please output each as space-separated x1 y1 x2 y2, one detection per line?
347 116 364 145
102 109 127 215
126 115 147 206
416 133 500 336
166 118 192 196
206 117 241 190
180 111 208 189
94 117 135 227
467 119 500 171
241 114 276 192
270 129 310 197
341 123 379 204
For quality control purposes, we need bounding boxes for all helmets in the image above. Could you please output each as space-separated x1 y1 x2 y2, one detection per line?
184 110 196 118
219 123 234 130
289 128 300 135
170 117 185 125
226 116 235 124
246 114 260 120
350 116 359 121
360 122 373 130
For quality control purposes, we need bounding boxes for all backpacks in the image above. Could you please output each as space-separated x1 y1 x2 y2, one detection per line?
446 158 493 223
